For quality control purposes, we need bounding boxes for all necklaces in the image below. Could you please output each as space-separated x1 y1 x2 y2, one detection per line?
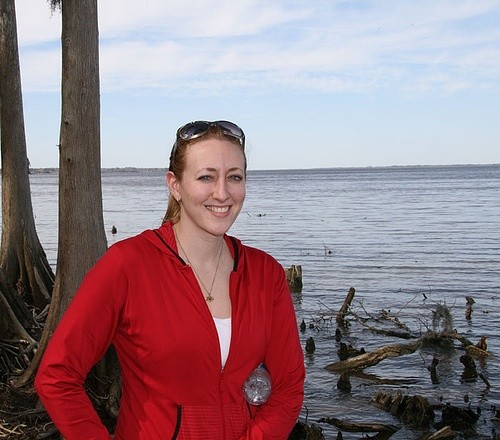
171 228 226 302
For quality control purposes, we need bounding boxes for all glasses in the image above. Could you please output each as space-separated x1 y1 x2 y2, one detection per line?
171 120 246 164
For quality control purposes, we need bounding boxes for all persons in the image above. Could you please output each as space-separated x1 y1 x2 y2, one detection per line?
33 119 306 440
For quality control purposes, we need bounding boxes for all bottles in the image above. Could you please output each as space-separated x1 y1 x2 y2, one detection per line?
242 363 272 406
